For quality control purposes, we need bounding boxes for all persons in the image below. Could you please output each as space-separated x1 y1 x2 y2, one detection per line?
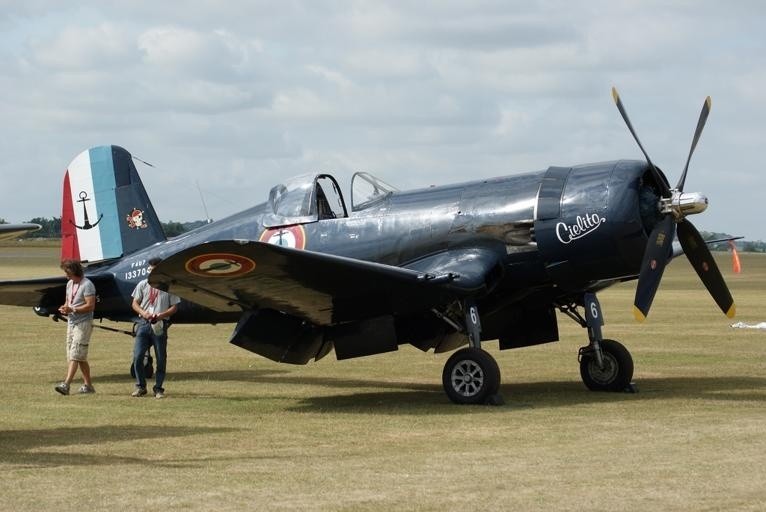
53 259 97 396
128 257 183 400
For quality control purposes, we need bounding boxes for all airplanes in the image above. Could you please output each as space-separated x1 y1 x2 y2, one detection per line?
0 85 745 407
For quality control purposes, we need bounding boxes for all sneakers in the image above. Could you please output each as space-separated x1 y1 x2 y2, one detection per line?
78 383 97 394
131 389 148 397
54 382 71 396
153 392 166 399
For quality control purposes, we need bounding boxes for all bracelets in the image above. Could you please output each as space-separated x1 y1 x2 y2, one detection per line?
73 306 77 313
